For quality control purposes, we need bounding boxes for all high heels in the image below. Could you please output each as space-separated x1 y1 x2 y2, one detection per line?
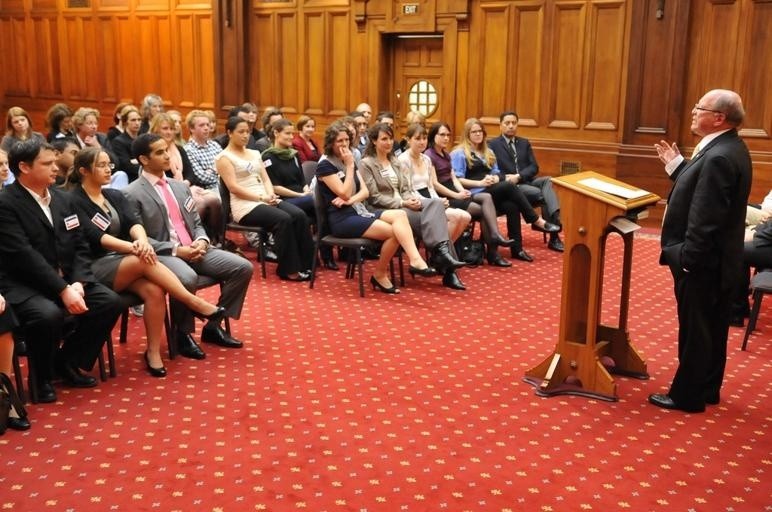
408 265 438 279
370 276 400 294
144 351 166 377
192 306 226 322
324 260 339 270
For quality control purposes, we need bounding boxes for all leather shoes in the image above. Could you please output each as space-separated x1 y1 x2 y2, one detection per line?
512 250 533 261
648 392 678 409
361 249 380 259
486 253 512 266
745 312 750 317
178 334 205 359
61 368 97 387
486 235 515 249
554 217 560 225
316 259 320 266
31 382 56 401
531 221 560 233
280 272 309 281
257 250 278 262
360 257 364 262
8 417 30 430
548 239 564 252
201 326 243 347
301 270 312 274
729 317 744 326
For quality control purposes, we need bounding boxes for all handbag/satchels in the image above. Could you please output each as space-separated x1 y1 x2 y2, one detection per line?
462 240 484 265
0 372 27 435
222 238 248 259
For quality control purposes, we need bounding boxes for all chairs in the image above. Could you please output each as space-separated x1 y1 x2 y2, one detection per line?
11 337 107 404
169 274 232 353
217 174 272 278
742 270 772 350
309 179 395 297
103 288 174 377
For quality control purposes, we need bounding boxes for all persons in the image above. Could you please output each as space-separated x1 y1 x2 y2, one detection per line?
108 103 133 142
243 103 264 141
728 189 772 326
0 150 9 191
121 135 253 358
150 114 222 248
423 123 515 266
488 113 563 253
184 110 278 264
316 125 438 293
2 107 46 153
397 124 471 274
167 111 186 148
351 112 371 157
139 95 163 134
113 106 143 182
215 108 257 150
261 120 340 271
291 115 321 165
356 103 372 131
1 295 30 429
0 140 119 404
376 113 400 155
216 117 315 281
47 105 75 153
310 116 380 270
203 111 215 142
449 118 560 261
362 123 468 289
72 108 127 190
400 112 431 153
51 138 80 185
649 90 751 411
257 110 284 152
58 148 225 376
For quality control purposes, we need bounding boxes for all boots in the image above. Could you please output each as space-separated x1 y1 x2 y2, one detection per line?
429 240 467 274
442 272 465 290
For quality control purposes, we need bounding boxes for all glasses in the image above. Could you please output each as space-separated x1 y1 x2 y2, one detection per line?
437 133 451 137
471 130 483 134
695 103 721 114
97 163 115 169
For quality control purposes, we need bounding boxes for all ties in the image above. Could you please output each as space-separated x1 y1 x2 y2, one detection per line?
157 179 192 246
509 140 518 173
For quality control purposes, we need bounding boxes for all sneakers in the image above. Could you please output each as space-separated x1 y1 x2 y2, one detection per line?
131 304 145 316
